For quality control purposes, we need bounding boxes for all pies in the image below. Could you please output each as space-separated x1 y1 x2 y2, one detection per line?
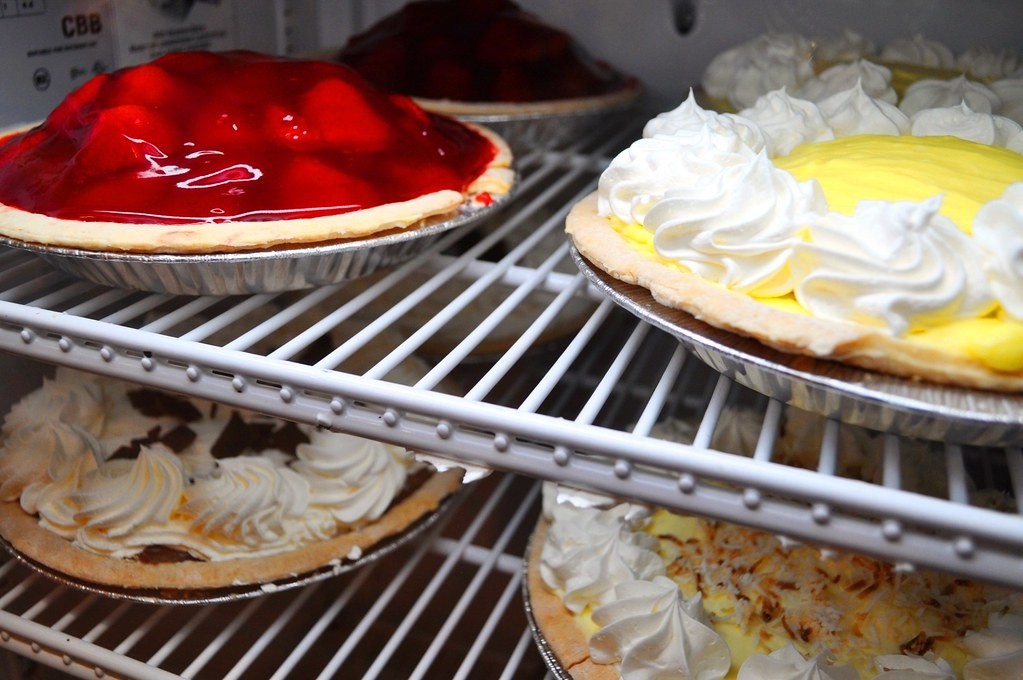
0 0 1023 680
